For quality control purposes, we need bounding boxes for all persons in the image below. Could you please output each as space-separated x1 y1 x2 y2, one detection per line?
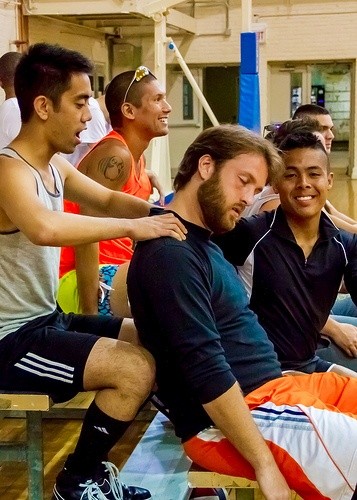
208 130 357 380
126 124 357 500
0 42 190 500
59 81 117 167
0 52 24 150
57 66 173 317
292 104 357 310
235 116 327 303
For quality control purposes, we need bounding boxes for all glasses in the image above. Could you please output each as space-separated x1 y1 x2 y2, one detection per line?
123 65 158 103
263 124 282 141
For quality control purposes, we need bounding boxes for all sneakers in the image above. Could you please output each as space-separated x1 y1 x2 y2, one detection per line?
52 469 109 500
63 453 151 500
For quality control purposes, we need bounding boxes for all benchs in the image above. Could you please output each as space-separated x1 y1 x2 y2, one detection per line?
0 391 53 500
188 462 262 499
